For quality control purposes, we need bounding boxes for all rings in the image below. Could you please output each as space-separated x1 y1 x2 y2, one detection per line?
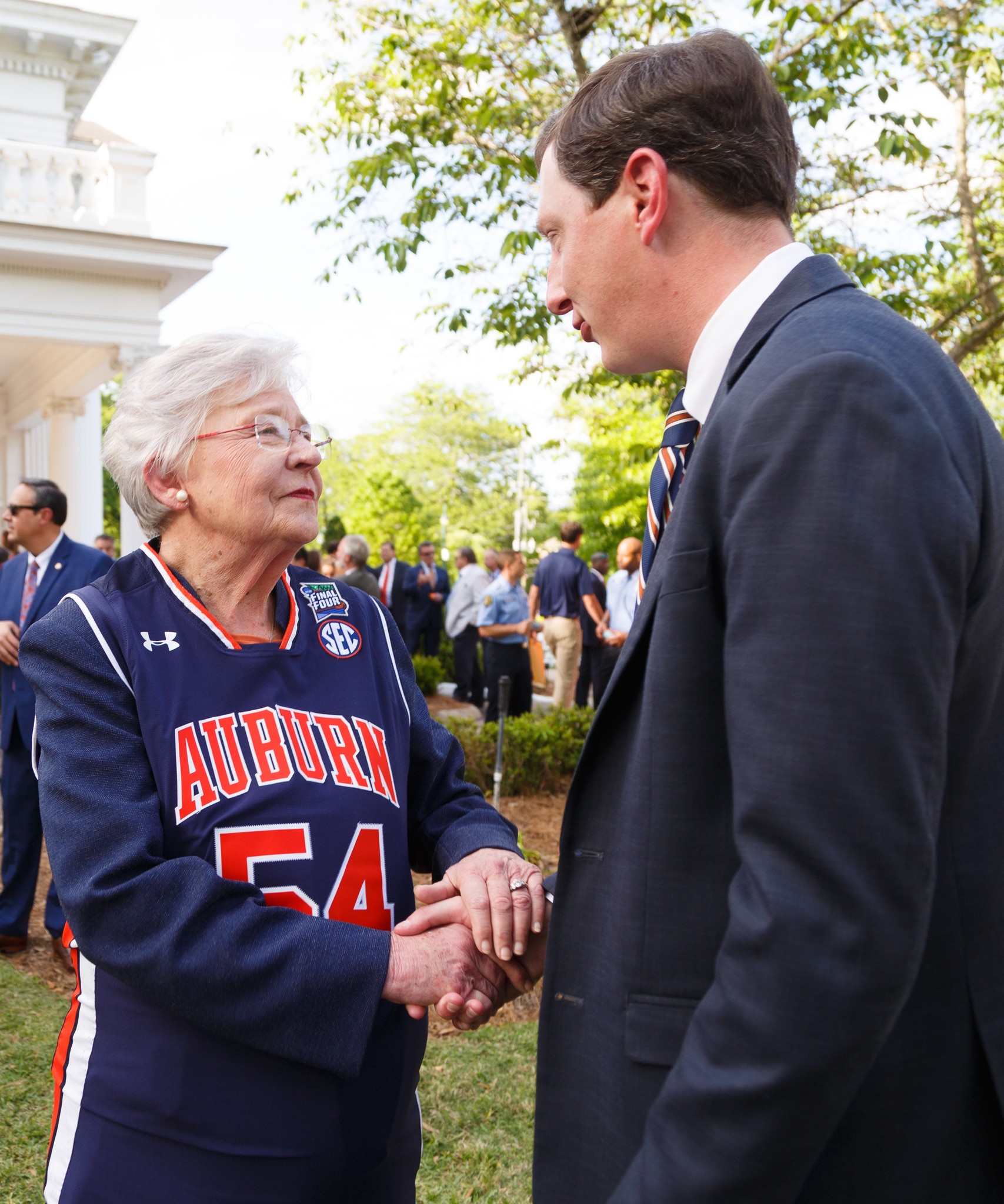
509 879 529 891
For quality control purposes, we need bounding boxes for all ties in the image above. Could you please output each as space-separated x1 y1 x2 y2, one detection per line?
427 564 435 588
19 560 41 631
635 387 702 617
380 564 389 606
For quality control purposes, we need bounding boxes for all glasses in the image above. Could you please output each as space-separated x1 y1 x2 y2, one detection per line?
191 414 334 460
5 504 35 517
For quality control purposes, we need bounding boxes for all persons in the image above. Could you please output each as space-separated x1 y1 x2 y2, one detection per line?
0 475 653 972
394 30 1004 1204
15 323 546 1204
